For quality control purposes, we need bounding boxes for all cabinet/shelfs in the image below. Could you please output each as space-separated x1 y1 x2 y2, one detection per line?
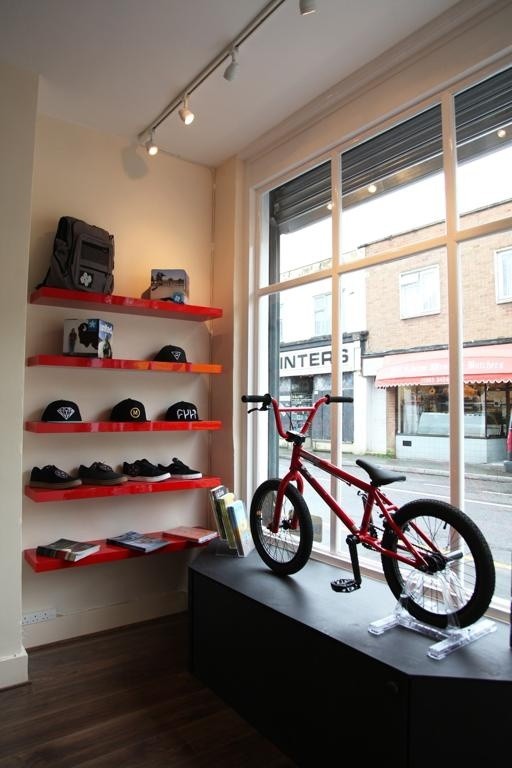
23 291 224 573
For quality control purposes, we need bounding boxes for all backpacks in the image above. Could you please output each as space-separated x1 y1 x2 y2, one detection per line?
35 216 114 294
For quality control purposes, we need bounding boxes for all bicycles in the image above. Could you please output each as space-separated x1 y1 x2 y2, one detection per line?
236 391 502 631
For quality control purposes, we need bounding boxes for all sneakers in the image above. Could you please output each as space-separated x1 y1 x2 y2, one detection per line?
124 459 171 482
30 465 82 489
158 458 202 480
78 462 128 485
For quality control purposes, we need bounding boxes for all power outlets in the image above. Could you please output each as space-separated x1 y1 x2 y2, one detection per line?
22 609 48 625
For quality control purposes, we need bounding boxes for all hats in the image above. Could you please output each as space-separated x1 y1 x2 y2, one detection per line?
166 401 200 421
41 401 84 422
111 398 151 422
154 345 186 362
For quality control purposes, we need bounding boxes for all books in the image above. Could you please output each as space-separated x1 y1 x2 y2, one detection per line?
106 531 171 552
163 526 217 544
36 538 101 562
209 485 256 556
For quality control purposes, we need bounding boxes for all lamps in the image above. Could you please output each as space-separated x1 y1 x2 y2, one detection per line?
137 0 318 157
